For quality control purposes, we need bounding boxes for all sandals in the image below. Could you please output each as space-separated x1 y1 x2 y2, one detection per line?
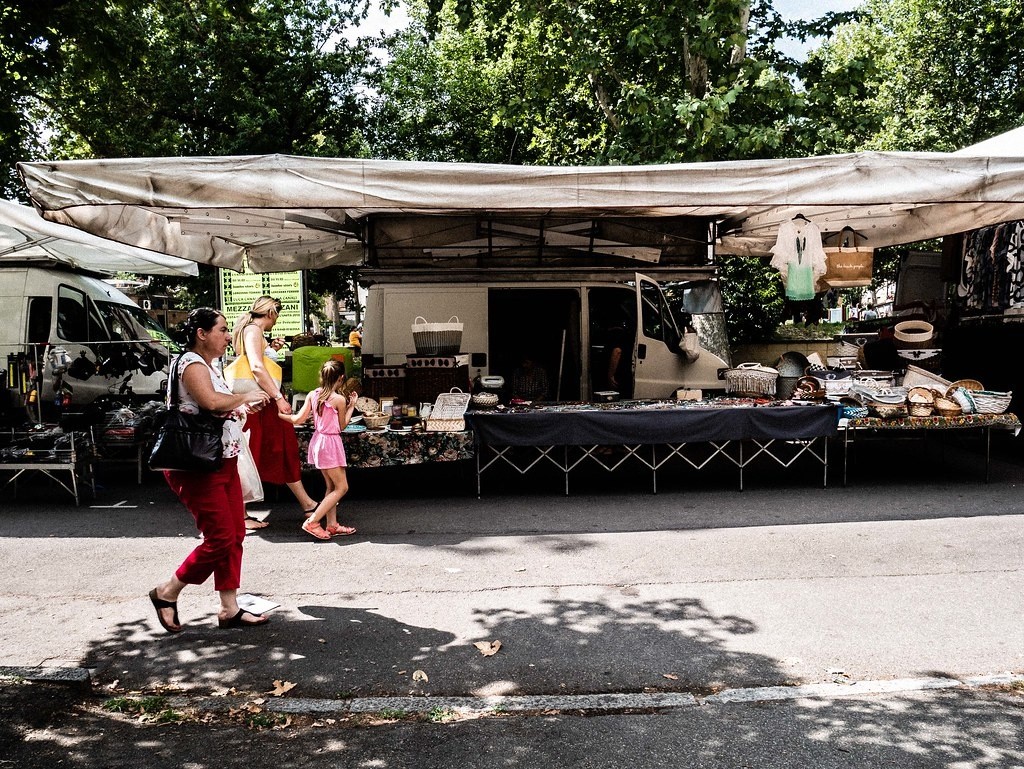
301 519 331 539
325 523 356 536
304 501 339 519
244 516 269 530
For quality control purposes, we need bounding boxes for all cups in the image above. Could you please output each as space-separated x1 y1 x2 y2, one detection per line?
392 404 417 416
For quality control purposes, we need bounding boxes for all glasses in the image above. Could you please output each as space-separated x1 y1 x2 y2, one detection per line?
273 298 281 304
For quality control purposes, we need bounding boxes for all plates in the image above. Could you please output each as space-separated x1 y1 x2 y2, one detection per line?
342 425 367 433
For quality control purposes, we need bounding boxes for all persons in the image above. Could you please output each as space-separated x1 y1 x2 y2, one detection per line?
148 310 271 633
863 304 877 320
349 325 363 349
232 299 322 529
278 360 358 539
599 324 630 397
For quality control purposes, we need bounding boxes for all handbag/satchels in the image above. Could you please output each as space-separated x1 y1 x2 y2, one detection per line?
822 226 874 286
144 352 228 471
223 325 282 400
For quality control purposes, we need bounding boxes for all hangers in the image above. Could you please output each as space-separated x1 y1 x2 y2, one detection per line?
825 216 868 242
778 208 816 230
966 218 1024 235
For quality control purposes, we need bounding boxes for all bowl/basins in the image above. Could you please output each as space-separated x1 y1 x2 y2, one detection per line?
362 411 391 429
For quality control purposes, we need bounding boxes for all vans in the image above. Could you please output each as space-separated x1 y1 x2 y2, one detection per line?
360 270 731 405
0 266 184 462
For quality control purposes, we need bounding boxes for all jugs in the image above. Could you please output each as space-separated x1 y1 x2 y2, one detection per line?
419 402 434 419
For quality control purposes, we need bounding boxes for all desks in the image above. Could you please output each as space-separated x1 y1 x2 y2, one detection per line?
462 398 839 503
287 425 474 473
404 353 469 400
837 411 1022 484
96 440 146 484
0 459 95 508
363 365 405 401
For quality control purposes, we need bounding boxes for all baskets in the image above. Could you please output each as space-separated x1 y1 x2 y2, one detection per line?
363 411 390 429
411 315 464 356
797 368 1012 420
473 393 498 408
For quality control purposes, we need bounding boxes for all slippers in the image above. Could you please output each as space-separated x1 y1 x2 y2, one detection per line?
218 607 270 627
149 588 184 632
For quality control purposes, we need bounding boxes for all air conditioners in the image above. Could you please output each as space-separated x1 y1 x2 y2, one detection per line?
138 299 151 310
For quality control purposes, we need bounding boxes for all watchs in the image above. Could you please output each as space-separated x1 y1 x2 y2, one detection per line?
275 393 282 401
